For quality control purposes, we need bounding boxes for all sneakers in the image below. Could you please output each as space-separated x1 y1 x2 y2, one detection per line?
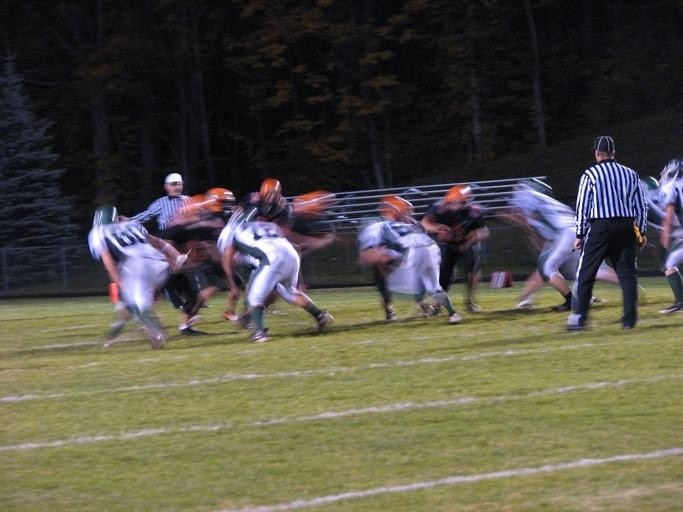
315 310 335 334
224 310 240 322
247 329 271 342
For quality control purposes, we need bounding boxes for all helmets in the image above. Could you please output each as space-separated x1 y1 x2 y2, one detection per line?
259 179 281 206
203 188 236 218
94 205 119 224
445 186 468 213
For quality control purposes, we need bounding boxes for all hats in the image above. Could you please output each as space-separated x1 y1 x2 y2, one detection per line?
590 136 615 152
165 173 183 183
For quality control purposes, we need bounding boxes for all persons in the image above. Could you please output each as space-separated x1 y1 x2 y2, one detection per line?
88 174 346 348
566 136 681 331
356 179 644 321
